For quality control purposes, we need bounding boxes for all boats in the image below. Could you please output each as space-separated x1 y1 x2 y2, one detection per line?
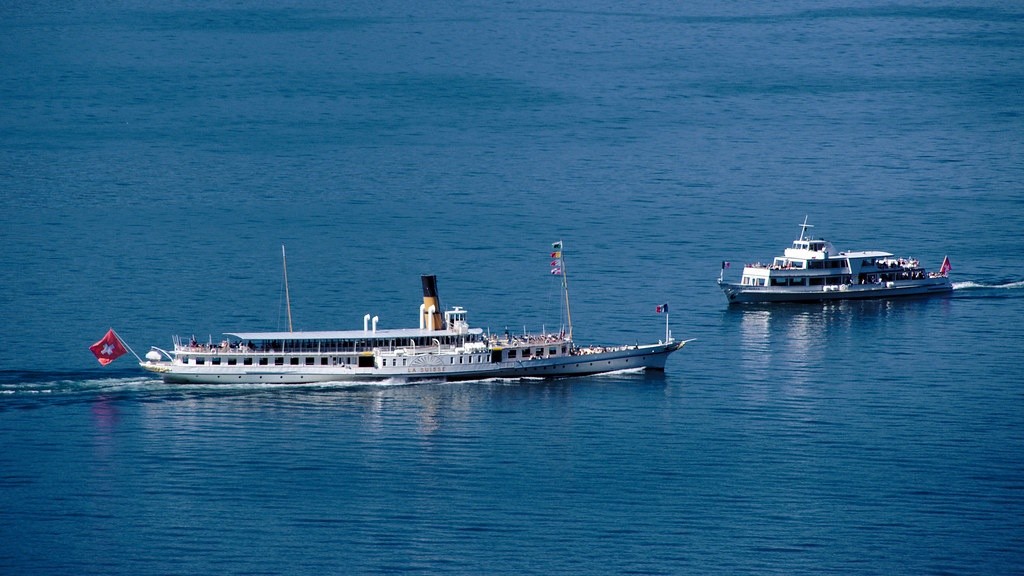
717 214 953 305
139 245 696 386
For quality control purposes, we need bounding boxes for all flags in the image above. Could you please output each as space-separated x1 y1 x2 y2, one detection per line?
657 304 667 313
88 329 127 367
551 252 560 258
724 262 730 268
550 261 560 266
551 268 560 274
552 242 562 250
941 257 951 272
